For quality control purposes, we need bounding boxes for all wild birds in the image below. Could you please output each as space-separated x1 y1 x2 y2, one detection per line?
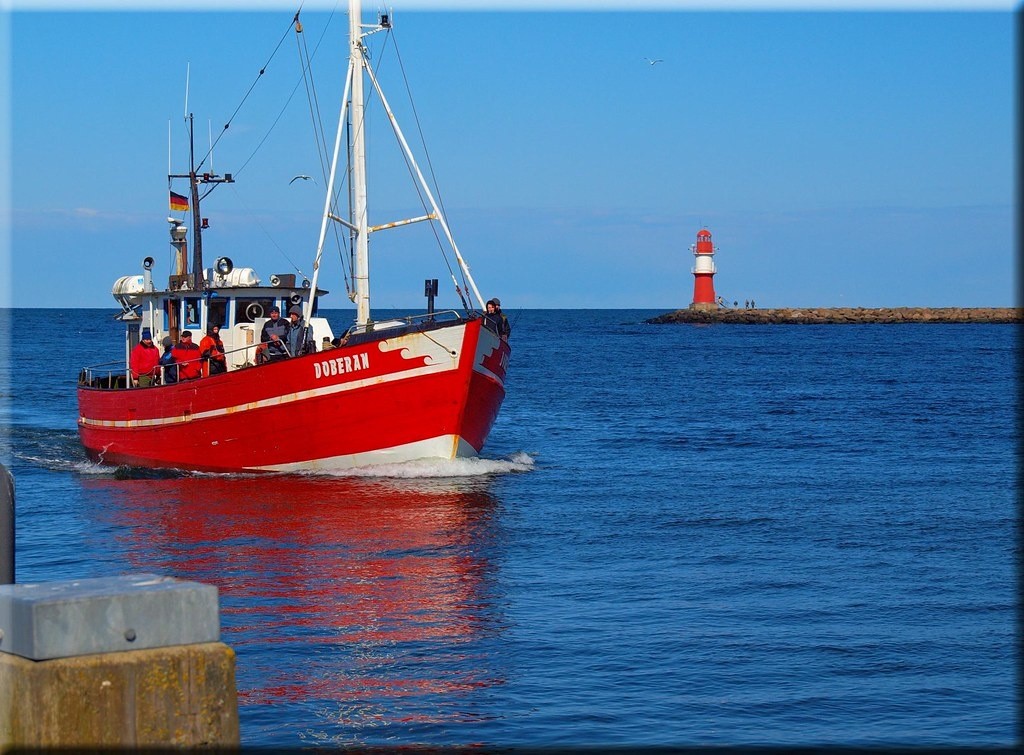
289 175 318 187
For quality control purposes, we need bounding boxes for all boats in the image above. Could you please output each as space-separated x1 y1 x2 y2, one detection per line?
75 0 513 474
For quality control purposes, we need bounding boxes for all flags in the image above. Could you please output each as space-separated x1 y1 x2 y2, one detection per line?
169 190 190 211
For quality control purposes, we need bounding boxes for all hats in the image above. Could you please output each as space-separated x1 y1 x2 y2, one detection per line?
340 329 352 338
142 331 151 340
181 331 192 338
163 336 172 346
270 306 280 313
493 298 500 305
289 305 302 317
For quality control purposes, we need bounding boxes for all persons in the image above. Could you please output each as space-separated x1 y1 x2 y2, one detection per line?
482 300 503 338
331 329 351 348
171 330 202 381
718 296 723 304
491 297 511 342
199 321 227 375
261 306 290 359
285 305 314 359
751 300 755 308
159 335 177 383
129 326 161 387
745 299 750 309
733 301 738 309
